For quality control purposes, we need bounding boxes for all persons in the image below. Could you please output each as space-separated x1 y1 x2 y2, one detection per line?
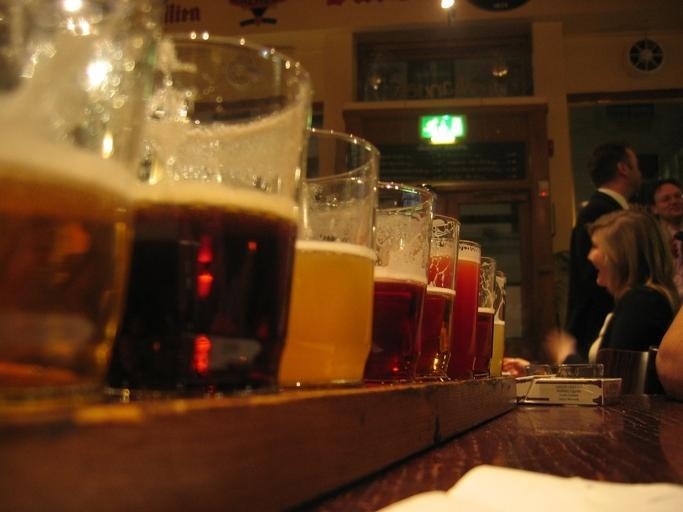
550 210 672 390
654 300 681 388
564 140 641 367
649 177 682 292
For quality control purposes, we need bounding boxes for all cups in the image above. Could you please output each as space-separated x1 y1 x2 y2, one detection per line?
527 364 551 374
489 270 506 379
473 256 496 380
362 179 438 385
555 362 604 378
108 29 315 397
415 213 462 382
446 239 482 380
0 0 165 409
278 128 381 388
